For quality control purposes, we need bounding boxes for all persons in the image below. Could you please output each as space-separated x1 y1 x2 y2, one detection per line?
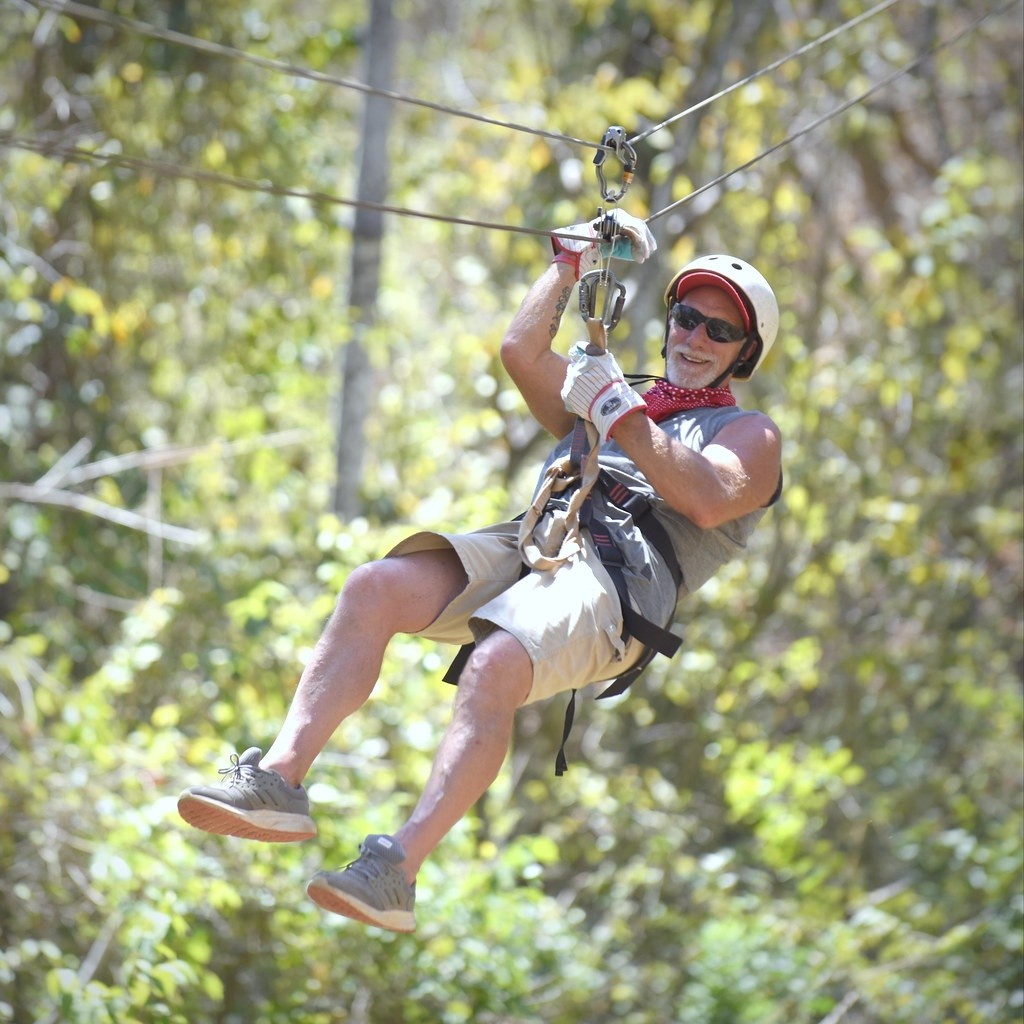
178 207 785 935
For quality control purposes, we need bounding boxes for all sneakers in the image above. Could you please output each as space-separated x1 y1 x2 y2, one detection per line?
308 835 418 932
177 747 317 842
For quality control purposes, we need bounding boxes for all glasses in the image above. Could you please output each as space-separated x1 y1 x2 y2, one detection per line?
668 301 748 344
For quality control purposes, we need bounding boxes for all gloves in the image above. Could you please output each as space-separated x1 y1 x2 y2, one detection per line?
550 208 657 278
560 343 648 441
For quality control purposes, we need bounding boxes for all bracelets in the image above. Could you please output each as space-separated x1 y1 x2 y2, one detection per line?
551 254 578 266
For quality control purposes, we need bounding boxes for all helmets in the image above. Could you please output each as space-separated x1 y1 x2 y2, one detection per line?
664 253 780 380
